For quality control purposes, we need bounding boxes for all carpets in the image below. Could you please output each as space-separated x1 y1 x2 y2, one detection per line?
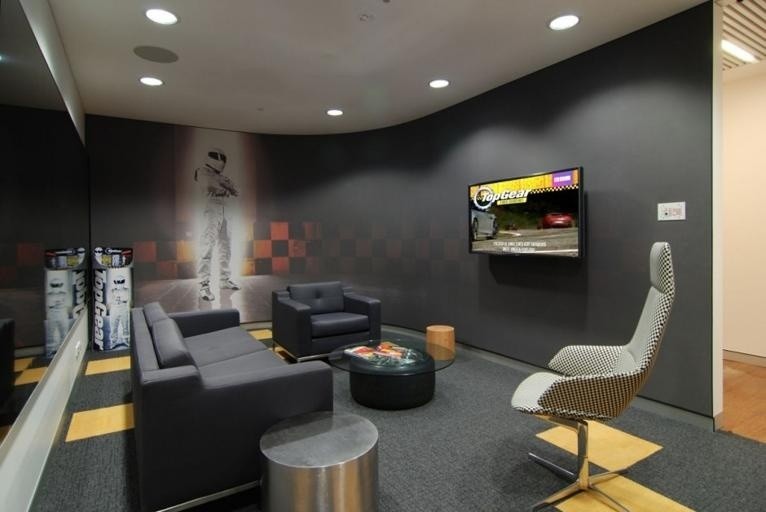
13 354 50 414
29 326 765 511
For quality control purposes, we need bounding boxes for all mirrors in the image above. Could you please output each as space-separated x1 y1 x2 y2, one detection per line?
0 0 93 469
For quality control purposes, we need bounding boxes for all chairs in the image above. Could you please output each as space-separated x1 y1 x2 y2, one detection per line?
510 242 674 512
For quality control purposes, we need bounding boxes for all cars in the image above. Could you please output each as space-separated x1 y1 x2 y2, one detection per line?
471 208 498 241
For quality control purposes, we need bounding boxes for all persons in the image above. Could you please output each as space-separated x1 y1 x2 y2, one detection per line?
193 147 241 301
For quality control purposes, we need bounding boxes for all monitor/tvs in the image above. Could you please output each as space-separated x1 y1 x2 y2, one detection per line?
467 166 587 263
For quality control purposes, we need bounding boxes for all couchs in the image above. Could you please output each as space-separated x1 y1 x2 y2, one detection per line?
271 280 383 363
130 302 333 512
0 318 16 418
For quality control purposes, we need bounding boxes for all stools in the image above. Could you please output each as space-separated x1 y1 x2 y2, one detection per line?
426 326 455 361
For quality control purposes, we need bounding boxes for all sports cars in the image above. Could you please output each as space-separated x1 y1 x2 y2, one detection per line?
536 213 573 229
